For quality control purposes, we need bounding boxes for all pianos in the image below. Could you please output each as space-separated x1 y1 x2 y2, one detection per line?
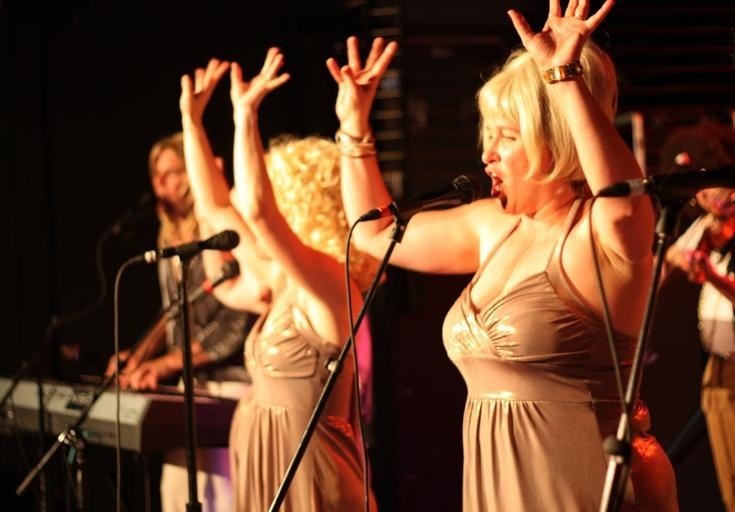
1 378 238 455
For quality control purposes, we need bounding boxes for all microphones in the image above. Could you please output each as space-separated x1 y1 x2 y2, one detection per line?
358 173 484 222
597 161 735 197
170 259 240 319
157 230 239 258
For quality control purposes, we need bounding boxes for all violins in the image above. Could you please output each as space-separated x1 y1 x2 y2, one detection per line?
685 191 735 282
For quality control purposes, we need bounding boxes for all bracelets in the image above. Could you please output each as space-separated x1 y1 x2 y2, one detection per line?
539 60 585 84
331 130 377 158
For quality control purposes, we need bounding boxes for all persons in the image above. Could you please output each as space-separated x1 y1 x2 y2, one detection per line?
327 0 680 511
180 47 380 511
104 131 261 511
668 165 735 511
